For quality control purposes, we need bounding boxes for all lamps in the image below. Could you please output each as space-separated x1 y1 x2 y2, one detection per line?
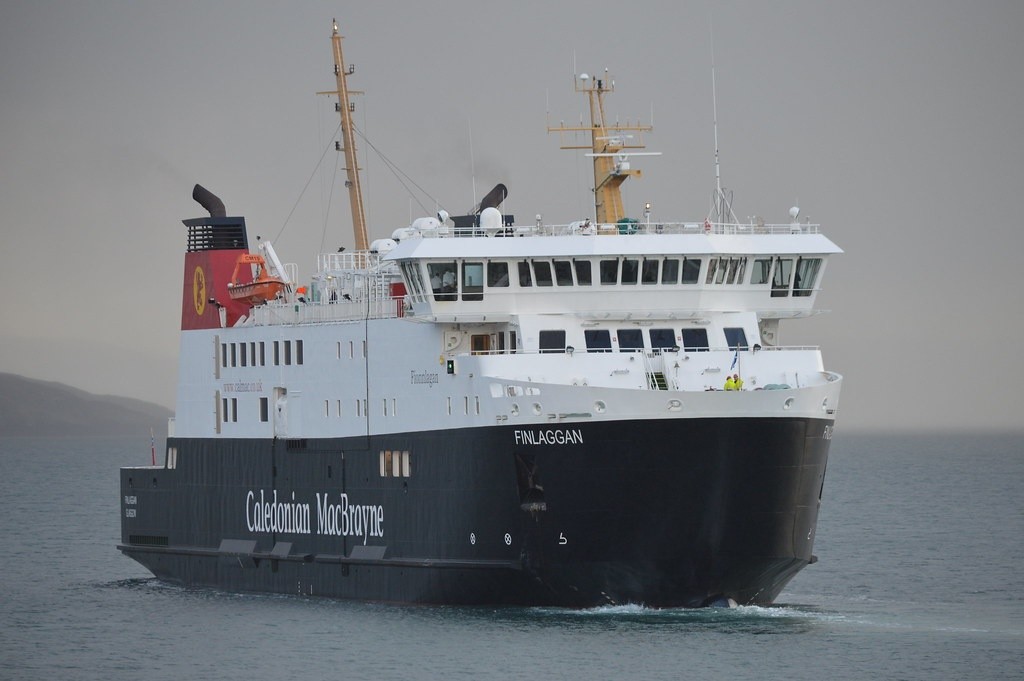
672 344 680 354
437 209 449 233
788 206 800 230
753 343 761 354
567 346 574 356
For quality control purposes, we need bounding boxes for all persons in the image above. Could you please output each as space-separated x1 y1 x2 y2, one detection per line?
724 373 744 391
788 269 801 296
431 268 455 301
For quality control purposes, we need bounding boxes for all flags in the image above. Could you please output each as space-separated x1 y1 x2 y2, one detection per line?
730 346 739 370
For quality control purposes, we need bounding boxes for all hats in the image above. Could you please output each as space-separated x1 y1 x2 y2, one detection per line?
727 376 731 380
733 374 738 377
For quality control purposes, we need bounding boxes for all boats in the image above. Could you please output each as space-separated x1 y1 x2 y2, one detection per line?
118 19 844 610
